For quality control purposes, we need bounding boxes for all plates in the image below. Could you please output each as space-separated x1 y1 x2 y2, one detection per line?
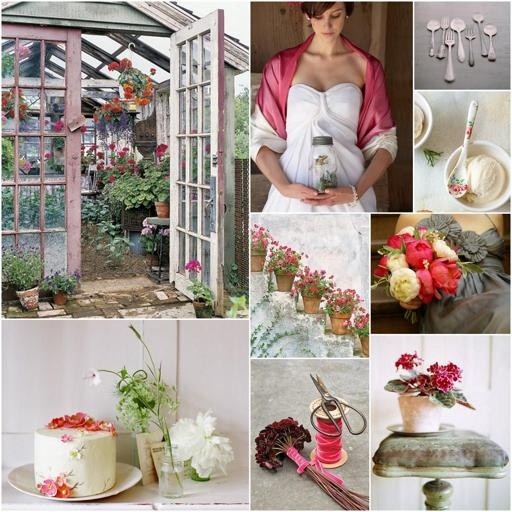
5 461 143 501
385 421 458 437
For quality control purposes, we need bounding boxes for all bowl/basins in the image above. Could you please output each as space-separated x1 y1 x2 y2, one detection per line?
414 90 434 151
443 141 511 212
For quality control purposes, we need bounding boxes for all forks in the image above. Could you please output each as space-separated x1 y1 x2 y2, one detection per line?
444 29 456 82
463 23 476 67
436 16 450 59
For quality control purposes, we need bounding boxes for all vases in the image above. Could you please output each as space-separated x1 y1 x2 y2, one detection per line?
52 291 69 306
397 395 446 434
14 286 41 312
193 298 212 317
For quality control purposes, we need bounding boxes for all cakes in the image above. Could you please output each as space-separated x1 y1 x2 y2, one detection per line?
33 412 118 498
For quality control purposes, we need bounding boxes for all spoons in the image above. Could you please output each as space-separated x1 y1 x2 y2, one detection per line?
426 19 441 57
445 99 480 199
472 11 488 56
449 16 467 63
483 23 498 61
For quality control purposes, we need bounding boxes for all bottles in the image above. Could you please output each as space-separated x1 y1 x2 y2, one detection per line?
307 135 338 195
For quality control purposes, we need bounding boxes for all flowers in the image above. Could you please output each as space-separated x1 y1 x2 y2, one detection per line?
38 263 82 297
251 416 369 511
34 322 236 500
2 42 212 188
384 349 479 411
250 220 368 358
184 259 217 318
370 225 483 325
1 239 45 294
138 217 170 242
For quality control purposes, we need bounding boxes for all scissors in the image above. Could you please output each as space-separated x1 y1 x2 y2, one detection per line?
309 373 367 437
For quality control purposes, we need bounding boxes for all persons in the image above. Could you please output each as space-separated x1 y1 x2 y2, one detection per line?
252 2 398 211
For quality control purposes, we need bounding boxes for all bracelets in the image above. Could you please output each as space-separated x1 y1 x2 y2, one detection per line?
347 184 358 208
